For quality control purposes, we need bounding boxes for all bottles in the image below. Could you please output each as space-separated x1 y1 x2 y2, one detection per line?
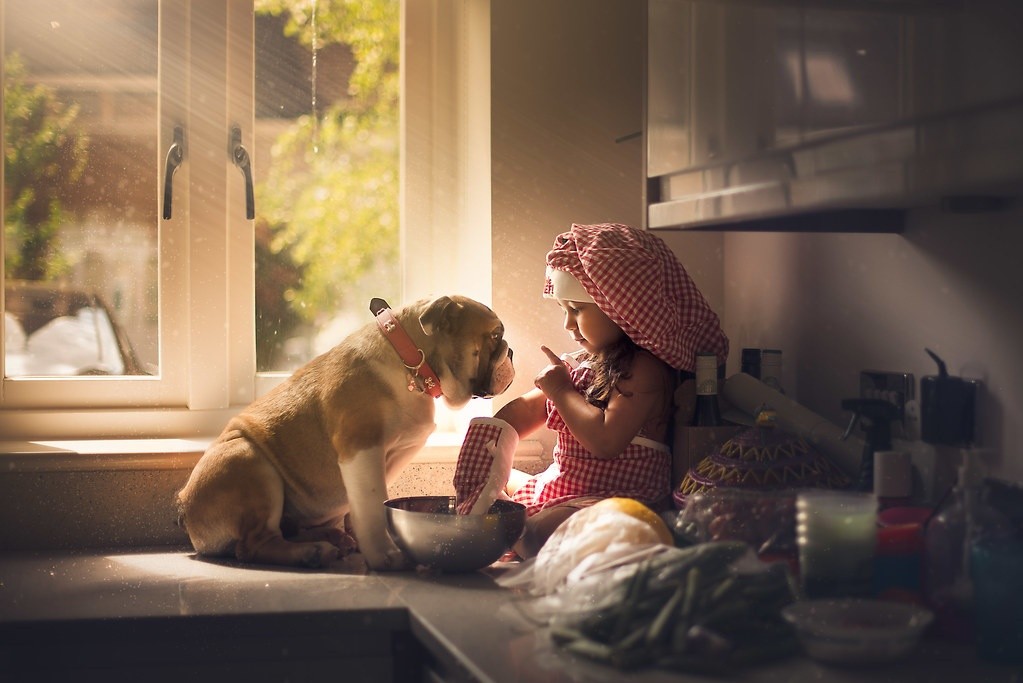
690 352 723 426
739 348 789 401
925 486 1014 644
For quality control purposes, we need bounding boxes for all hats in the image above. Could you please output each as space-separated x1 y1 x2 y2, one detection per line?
543 265 598 304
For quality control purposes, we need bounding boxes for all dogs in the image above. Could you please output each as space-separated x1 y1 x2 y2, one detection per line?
177 295 515 573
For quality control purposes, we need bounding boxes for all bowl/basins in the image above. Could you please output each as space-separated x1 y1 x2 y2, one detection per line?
781 595 934 664
381 495 526 574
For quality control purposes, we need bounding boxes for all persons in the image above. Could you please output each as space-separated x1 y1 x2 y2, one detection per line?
454 222 728 562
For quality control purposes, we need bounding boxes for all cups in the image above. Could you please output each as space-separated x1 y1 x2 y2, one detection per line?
795 488 878 602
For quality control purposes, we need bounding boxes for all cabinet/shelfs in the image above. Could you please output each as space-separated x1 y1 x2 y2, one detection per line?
639 0 1023 236
